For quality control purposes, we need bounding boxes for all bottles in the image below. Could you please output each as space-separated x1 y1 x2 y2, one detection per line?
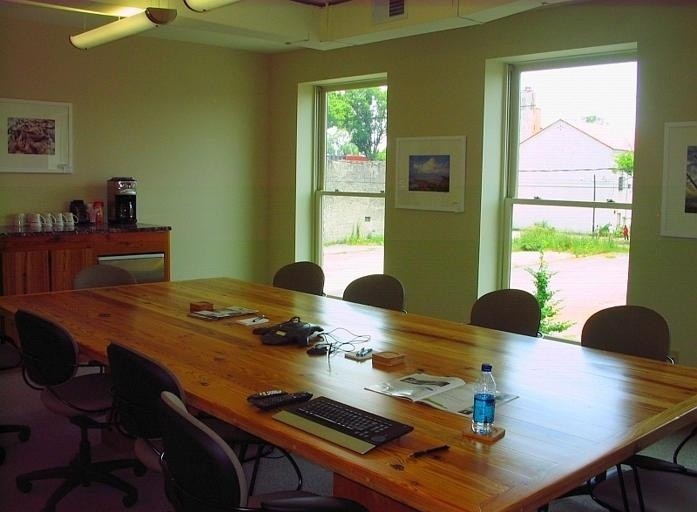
471 362 497 434
70 200 104 225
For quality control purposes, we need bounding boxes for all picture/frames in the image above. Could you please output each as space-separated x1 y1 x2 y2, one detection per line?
0 97 74 175
393 135 466 213
660 120 696 241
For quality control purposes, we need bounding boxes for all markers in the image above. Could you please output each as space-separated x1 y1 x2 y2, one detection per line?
356 348 363 355
253 316 262 322
361 348 372 356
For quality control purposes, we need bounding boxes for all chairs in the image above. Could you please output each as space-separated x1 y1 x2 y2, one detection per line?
71 266 137 292
274 262 324 297
589 413 697 512
580 305 674 367
343 274 405 312
469 288 542 338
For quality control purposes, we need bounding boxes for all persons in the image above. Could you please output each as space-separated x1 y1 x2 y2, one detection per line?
624 224 630 240
608 223 615 242
594 224 601 239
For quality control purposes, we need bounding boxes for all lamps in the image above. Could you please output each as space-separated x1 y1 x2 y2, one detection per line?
184 0 236 12
68 1 177 52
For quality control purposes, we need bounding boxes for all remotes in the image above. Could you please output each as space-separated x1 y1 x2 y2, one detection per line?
246 389 288 402
253 391 313 410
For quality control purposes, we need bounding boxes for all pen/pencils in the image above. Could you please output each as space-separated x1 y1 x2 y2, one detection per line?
408 444 450 459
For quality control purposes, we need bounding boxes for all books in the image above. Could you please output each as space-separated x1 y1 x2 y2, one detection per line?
365 373 520 419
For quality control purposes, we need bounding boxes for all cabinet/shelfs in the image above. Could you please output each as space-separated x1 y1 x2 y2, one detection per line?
0 222 170 292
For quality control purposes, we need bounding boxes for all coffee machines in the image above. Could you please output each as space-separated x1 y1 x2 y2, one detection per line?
108 177 138 226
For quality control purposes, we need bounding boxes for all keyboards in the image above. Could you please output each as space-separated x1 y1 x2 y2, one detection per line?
271 396 414 455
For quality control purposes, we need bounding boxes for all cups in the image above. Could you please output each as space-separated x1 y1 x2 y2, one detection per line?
16 212 78 227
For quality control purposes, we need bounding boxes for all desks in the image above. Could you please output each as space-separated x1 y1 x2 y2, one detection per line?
0 278 697 512
